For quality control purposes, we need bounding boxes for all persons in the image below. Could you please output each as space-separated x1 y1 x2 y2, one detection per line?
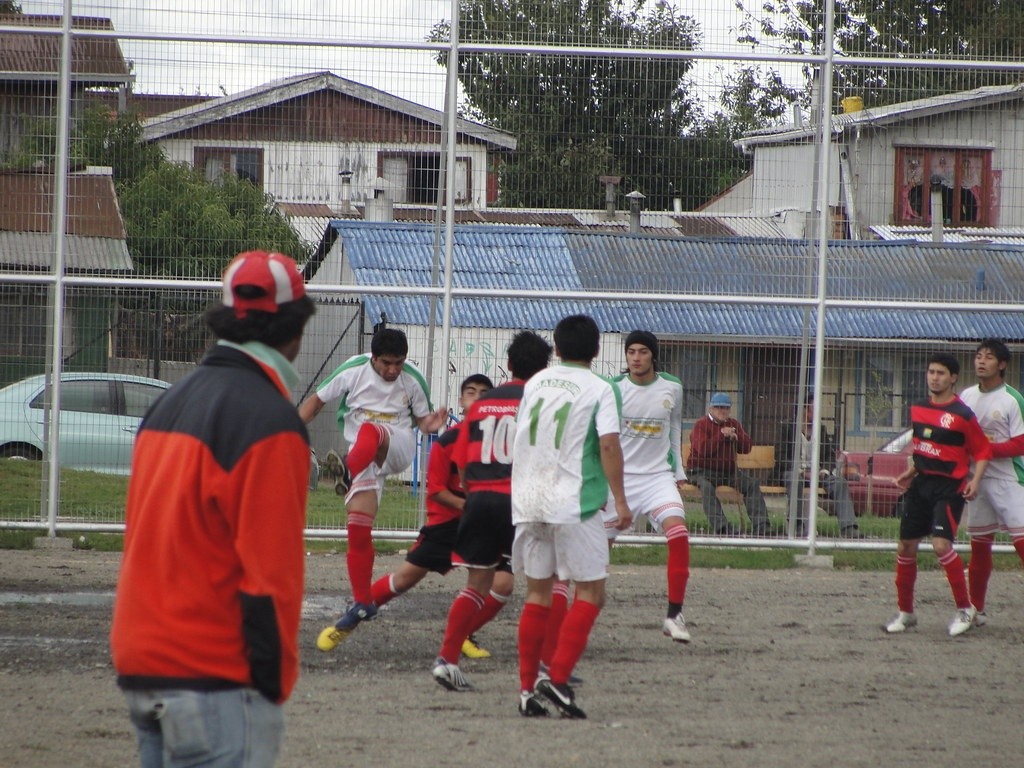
886 355 993 637
685 392 778 537
316 373 511 659
597 330 691 643
511 314 633 721
774 394 865 539
432 330 584 693
960 339 1024 627
298 329 451 621
108 251 316 768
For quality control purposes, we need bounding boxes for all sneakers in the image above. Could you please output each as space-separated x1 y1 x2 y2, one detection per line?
519 690 552 717
887 612 916 633
432 656 474 691
335 602 378 631
534 677 587 719
538 660 584 686
949 602 977 636
976 612 987 626
327 450 352 496
317 626 351 652
663 613 690 644
462 634 492 658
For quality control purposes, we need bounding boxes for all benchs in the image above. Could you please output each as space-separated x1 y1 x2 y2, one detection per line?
675 444 828 503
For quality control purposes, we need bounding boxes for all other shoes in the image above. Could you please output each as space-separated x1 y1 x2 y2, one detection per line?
754 526 775 535
841 526 864 539
795 524 805 536
718 526 739 535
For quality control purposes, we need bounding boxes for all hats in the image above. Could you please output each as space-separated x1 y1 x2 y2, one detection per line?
221 252 305 318
710 392 732 407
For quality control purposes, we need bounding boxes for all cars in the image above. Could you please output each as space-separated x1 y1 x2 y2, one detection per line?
829 426 919 518
0 371 320 493
